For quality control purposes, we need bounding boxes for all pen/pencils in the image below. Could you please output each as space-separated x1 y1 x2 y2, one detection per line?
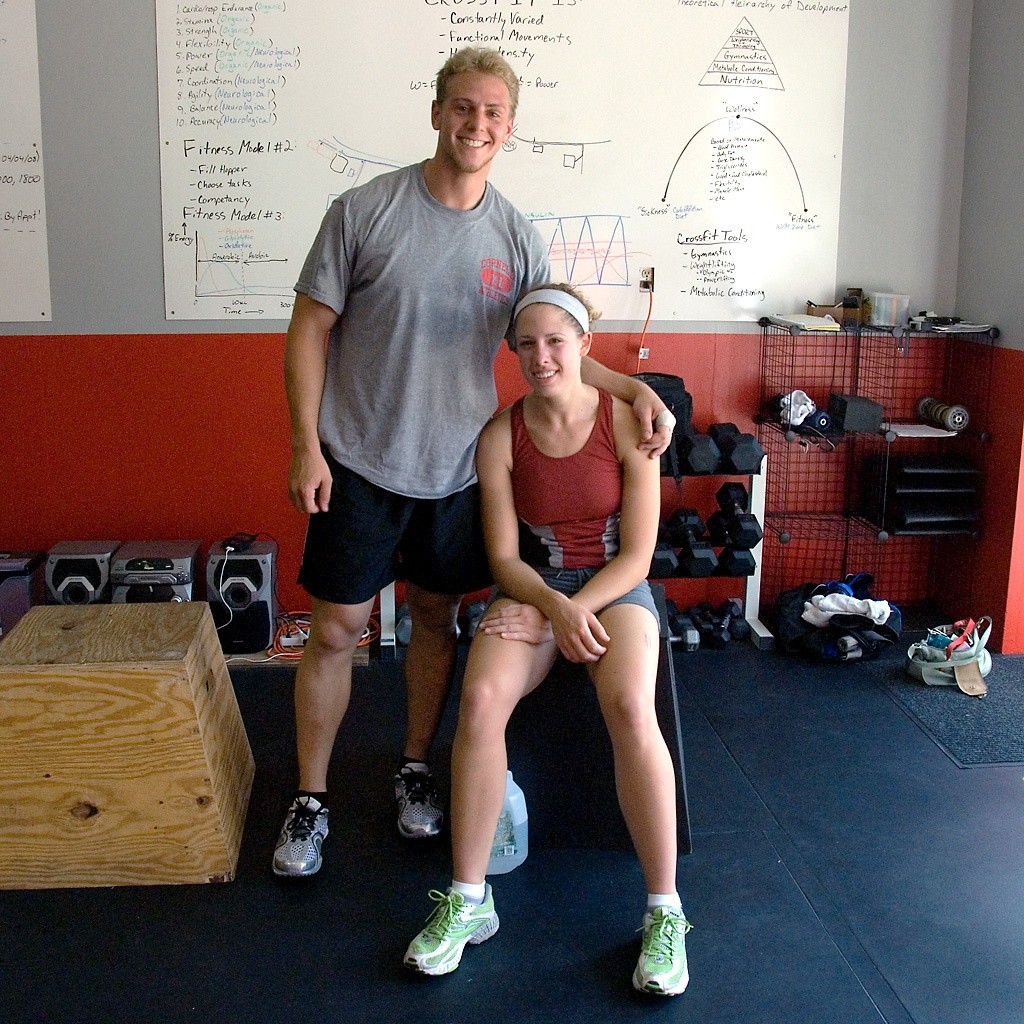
806 303 811 306
807 300 819 307
834 302 843 308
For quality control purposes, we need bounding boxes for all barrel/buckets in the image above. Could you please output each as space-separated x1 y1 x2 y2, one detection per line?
486 770 528 875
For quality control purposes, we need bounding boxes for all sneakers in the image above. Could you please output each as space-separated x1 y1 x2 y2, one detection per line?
404 882 500 975
392 762 443 837
632 906 694 998
273 795 330 875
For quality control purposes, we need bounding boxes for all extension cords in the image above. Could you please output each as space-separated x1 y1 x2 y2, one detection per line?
279 633 309 645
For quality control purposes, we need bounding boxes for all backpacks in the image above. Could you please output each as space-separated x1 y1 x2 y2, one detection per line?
776 569 901 659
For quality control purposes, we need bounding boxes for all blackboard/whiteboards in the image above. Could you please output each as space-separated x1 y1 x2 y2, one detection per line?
0 0 51 321
156 0 850 322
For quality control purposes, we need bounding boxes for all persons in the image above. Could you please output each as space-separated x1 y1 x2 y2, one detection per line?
403 283 693 997
270 47 675 875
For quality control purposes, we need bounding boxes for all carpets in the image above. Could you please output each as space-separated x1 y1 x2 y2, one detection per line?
853 651 1024 770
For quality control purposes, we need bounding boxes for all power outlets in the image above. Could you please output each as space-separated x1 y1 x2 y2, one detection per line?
638 266 656 293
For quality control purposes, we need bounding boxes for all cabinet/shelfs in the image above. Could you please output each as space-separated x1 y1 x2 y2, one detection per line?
646 452 775 653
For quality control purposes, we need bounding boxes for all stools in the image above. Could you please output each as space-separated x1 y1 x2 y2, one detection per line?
505 582 693 856
0 602 258 892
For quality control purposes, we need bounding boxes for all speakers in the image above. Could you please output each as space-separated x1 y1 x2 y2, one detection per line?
45 539 121 607
207 540 275 655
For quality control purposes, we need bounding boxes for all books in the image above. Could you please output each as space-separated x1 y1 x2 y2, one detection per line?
769 314 842 333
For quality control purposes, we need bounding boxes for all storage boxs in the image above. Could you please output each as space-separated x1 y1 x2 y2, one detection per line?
807 304 844 325
865 292 910 327
843 308 863 327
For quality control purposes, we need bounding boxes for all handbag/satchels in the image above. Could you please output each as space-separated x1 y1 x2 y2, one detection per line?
907 615 994 696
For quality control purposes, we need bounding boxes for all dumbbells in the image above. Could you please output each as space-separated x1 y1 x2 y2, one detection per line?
645 423 767 650
